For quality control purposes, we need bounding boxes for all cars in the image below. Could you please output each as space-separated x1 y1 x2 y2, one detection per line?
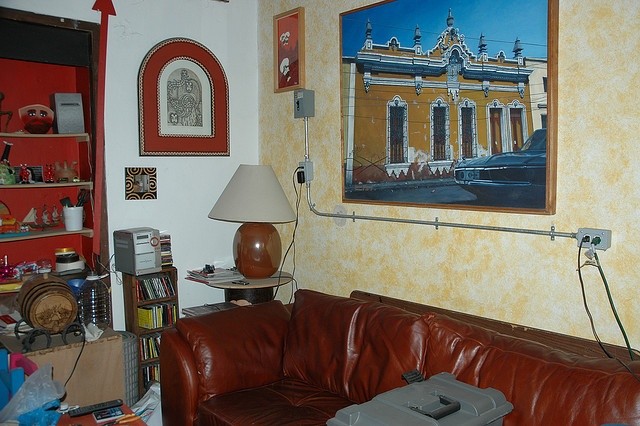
454 129 546 208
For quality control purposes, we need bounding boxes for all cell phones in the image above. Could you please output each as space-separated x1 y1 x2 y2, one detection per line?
231 280 250 285
92 406 126 424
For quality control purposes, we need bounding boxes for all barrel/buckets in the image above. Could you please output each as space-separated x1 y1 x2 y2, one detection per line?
18 273 78 335
78 270 112 330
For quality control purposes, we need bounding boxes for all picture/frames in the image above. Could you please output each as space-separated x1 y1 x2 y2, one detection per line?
125 167 157 201
273 6 306 94
339 1 560 216
138 37 230 157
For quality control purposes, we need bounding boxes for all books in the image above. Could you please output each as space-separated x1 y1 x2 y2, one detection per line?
161 235 173 267
142 361 160 387
136 275 176 302
140 331 162 361
137 301 177 330
185 267 244 284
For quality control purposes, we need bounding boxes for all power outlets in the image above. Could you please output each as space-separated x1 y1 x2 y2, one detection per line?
299 161 313 182
577 227 612 251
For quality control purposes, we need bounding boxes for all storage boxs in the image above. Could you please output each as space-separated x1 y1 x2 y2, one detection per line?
325 369 513 426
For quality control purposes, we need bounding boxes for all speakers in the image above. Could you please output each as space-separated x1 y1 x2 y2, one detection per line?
112 226 163 277
52 91 84 134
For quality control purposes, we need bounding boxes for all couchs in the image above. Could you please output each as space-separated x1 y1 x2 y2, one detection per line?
160 290 640 426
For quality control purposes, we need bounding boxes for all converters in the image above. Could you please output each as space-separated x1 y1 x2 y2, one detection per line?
297 171 305 184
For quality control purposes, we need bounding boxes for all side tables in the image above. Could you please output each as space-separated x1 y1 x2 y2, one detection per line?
198 268 294 302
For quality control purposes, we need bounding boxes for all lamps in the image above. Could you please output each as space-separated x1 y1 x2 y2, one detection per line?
208 164 297 279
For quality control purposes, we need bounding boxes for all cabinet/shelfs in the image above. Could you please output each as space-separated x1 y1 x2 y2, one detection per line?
0 7 108 297
123 267 180 400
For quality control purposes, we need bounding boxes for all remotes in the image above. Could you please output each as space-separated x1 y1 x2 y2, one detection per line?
68 399 123 418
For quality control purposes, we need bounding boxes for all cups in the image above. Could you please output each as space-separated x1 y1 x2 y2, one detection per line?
63 206 84 231
